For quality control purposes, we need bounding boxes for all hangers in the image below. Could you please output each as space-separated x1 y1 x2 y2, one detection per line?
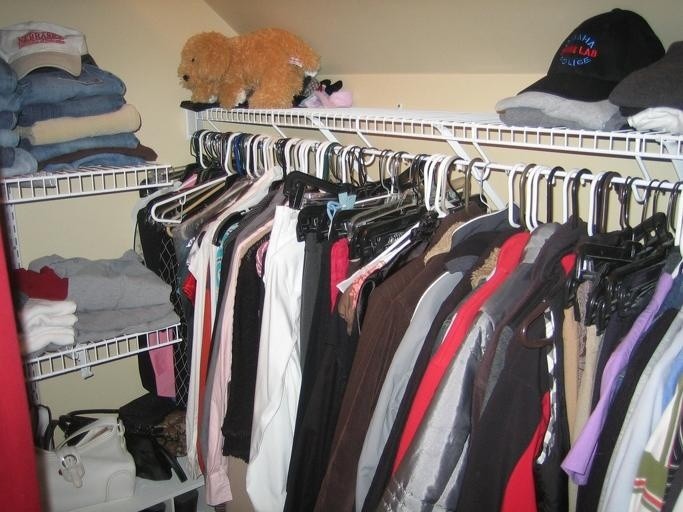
150 127 683 348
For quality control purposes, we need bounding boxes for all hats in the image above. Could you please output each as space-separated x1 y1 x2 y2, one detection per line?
0 21 91 82
517 6 665 103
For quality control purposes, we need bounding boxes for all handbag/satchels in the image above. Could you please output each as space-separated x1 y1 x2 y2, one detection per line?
35 416 137 512
59 413 188 483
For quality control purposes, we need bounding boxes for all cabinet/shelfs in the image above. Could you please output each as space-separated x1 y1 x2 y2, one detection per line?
2 157 182 386
